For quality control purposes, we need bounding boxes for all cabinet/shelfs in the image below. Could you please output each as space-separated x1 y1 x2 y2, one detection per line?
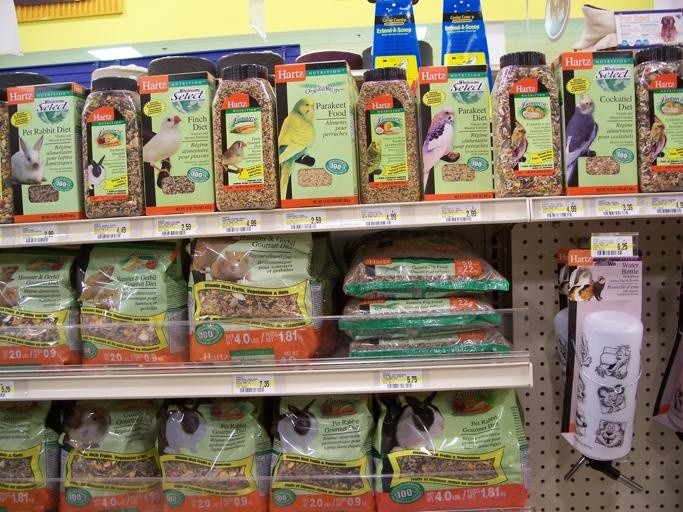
0 185 682 403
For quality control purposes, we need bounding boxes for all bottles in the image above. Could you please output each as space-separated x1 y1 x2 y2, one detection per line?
576 311 644 461
80 77 144 218
490 50 565 198
355 68 421 204
210 63 279 212
0 72 50 224
634 45 681 191
552 307 569 379
82 44 681 88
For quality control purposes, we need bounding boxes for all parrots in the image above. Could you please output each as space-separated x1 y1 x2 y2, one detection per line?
566 93 598 188
512 120 528 169
650 115 666 164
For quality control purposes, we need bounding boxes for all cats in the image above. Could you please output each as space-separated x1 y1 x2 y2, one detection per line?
88 155 106 183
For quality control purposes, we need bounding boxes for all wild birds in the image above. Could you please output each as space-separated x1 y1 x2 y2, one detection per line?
143 114 183 174
422 107 455 194
366 137 382 175
278 96 315 200
217 140 248 173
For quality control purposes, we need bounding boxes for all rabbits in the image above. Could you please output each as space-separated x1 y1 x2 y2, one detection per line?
11 134 44 184
